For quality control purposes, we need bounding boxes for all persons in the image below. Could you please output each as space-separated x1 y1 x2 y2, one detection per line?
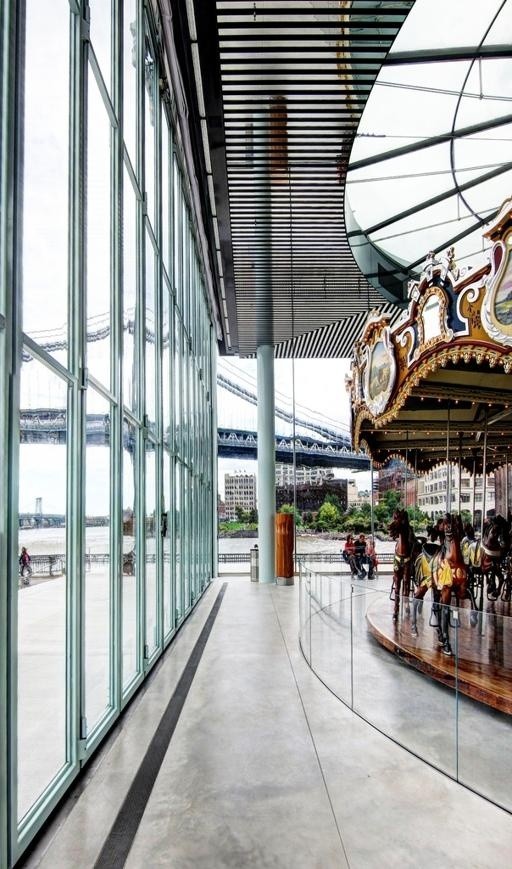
342 531 379 579
19 546 33 576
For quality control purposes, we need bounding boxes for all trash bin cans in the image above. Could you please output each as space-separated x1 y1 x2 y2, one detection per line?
250 544 259 582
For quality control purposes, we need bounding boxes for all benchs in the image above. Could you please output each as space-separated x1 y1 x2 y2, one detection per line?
363 560 394 579
